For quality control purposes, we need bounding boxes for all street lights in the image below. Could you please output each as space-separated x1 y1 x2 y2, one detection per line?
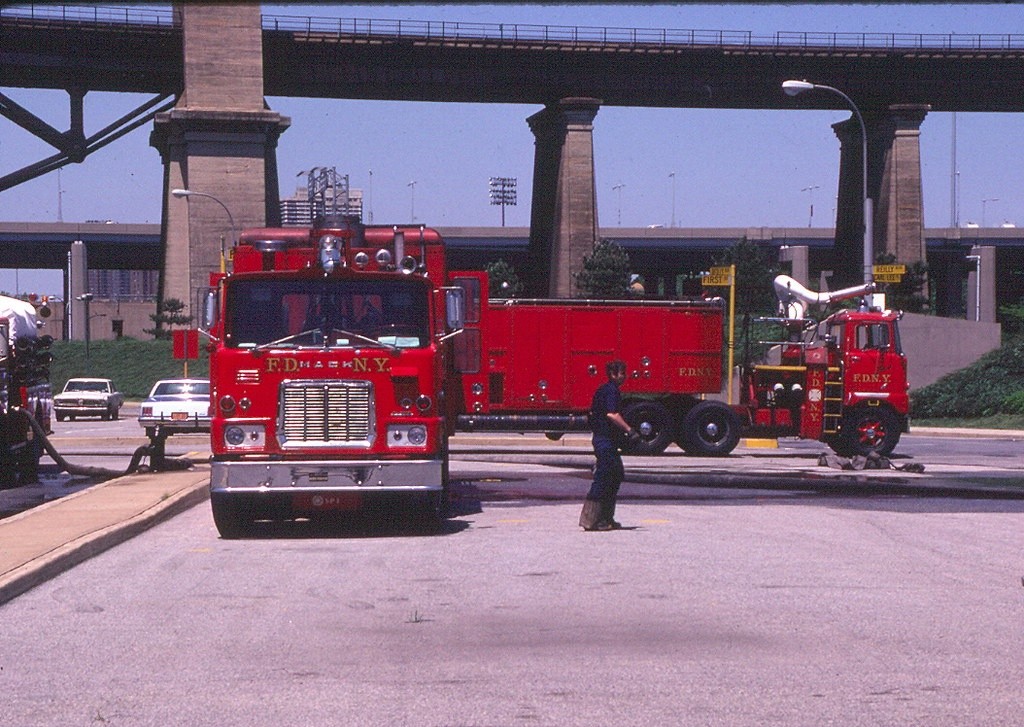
489 176 517 227
782 80 874 307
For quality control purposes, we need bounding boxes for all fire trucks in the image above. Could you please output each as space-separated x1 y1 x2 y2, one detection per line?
456 296 911 458
0 291 54 487
195 214 491 538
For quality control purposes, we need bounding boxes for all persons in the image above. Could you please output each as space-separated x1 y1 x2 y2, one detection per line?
579 360 631 530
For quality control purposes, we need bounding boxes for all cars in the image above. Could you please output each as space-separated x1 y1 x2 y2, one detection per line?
53 378 125 422
137 379 211 440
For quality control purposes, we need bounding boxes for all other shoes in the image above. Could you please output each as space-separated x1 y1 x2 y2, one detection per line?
585 518 620 530
